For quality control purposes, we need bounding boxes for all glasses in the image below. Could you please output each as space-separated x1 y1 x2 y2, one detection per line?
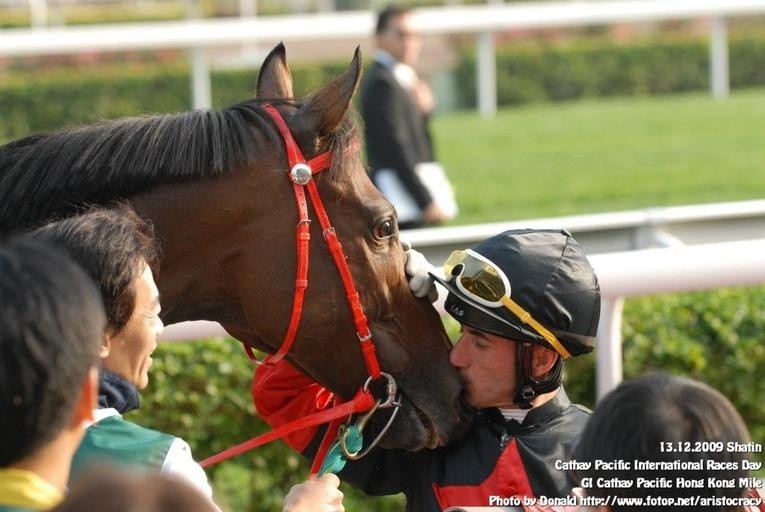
441 246 512 309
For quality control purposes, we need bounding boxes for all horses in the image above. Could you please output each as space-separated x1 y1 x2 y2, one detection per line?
0 41 475 452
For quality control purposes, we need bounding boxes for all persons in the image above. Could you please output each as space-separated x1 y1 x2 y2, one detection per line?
359 5 459 230
251 229 599 512
0 234 106 512
570 369 765 511
0 199 344 512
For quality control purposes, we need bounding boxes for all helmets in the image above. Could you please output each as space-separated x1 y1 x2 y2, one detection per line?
427 226 600 358
